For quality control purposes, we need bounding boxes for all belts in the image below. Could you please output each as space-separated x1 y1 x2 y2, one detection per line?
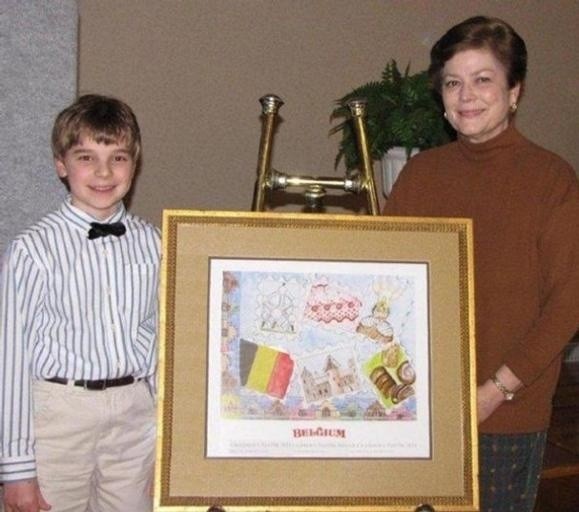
45 375 135 391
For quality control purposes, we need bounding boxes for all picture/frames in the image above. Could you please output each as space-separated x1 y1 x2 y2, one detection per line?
152 209 480 512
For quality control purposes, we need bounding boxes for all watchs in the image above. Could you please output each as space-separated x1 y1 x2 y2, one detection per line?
489 372 516 402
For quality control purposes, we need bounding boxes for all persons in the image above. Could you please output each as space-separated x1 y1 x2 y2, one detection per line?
376 13 578 512
1 95 162 511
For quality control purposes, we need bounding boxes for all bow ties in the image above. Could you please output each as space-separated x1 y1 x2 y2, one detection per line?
88 222 126 239
